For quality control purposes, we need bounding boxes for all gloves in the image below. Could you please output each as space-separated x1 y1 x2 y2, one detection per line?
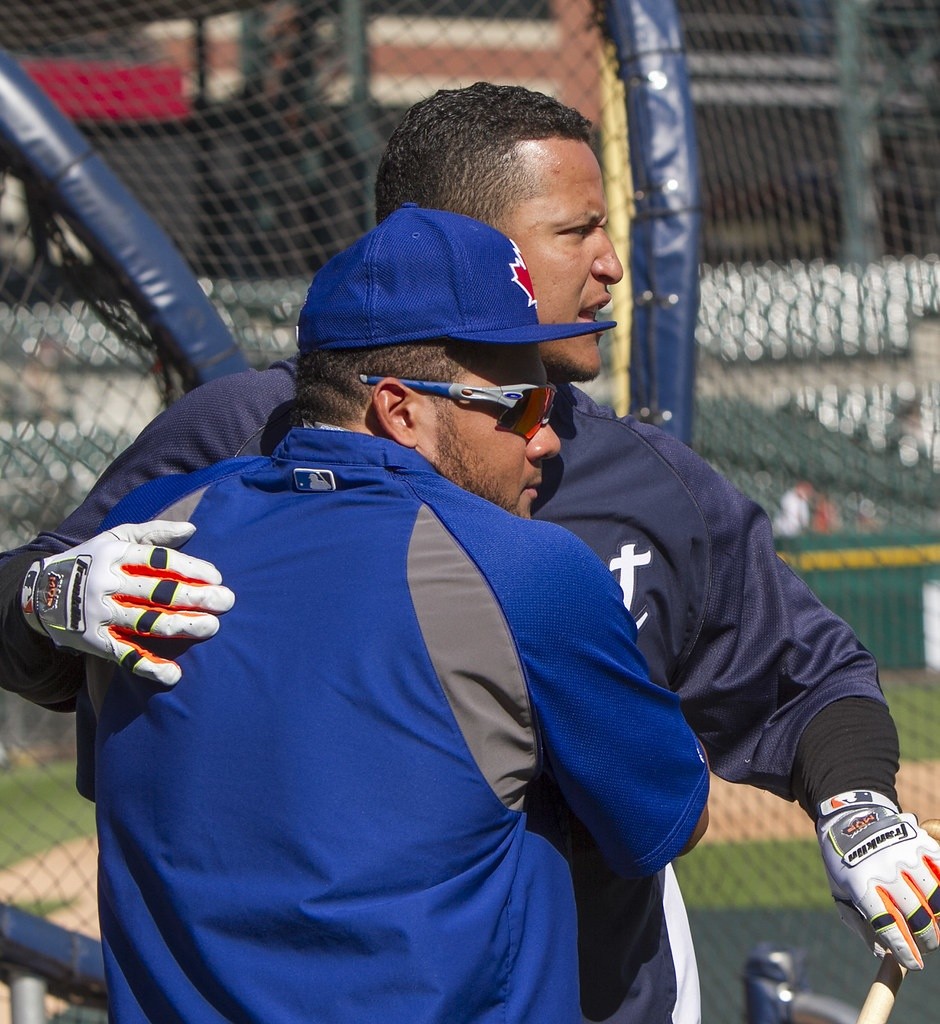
816 789 940 969
21 520 235 685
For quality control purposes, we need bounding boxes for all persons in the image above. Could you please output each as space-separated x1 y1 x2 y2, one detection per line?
80 201 711 1024
0 84 940 1024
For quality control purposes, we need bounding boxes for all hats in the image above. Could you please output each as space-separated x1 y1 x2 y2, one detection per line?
295 201 616 343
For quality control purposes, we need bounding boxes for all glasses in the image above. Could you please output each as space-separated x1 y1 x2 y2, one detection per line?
360 373 557 441
